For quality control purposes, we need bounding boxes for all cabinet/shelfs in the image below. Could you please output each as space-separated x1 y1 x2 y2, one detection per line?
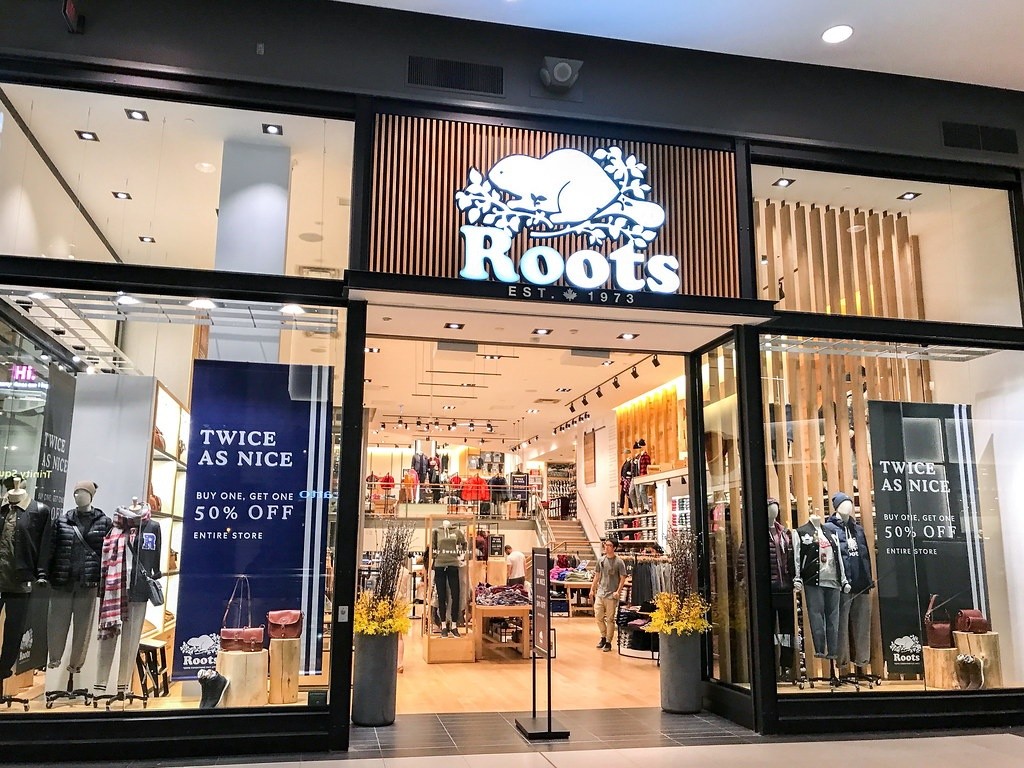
43 372 190 695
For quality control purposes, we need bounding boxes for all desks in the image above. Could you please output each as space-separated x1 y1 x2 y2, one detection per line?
470 603 531 661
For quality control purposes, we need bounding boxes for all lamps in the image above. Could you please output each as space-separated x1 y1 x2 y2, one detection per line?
365 342 659 452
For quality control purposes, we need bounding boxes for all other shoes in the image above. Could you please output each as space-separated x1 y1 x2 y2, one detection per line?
615 510 650 516
603 643 611 652
597 637 606 648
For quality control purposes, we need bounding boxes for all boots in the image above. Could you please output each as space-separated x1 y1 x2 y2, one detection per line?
198 669 229 708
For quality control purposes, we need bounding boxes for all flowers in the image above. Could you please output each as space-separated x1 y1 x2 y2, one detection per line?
351 523 415 636
642 522 713 636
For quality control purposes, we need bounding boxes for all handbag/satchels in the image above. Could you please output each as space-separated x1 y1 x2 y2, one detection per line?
169 550 177 570
242 628 264 651
154 426 166 449
955 609 982 630
965 617 987 632
147 577 163 606
220 628 250 650
147 484 162 511
268 610 302 638
924 619 953 648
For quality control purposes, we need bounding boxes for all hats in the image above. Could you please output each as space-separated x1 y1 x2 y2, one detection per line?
767 498 779 508
832 492 852 510
73 481 98 502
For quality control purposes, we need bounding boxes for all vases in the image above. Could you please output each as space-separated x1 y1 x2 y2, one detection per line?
659 631 703 713
355 629 398 728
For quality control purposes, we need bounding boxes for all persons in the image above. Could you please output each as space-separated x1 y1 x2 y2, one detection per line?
792 509 850 659
38 482 112 673
0 477 50 679
589 538 626 651
505 545 526 586
736 499 794 681
432 520 467 638
412 451 441 505
827 493 874 676
94 497 161 691
396 566 409 672
618 439 650 516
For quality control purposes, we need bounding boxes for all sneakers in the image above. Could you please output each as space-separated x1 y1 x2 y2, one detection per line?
967 656 984 689
442 629 450 638
451 628 460 638
958 657 970 689
